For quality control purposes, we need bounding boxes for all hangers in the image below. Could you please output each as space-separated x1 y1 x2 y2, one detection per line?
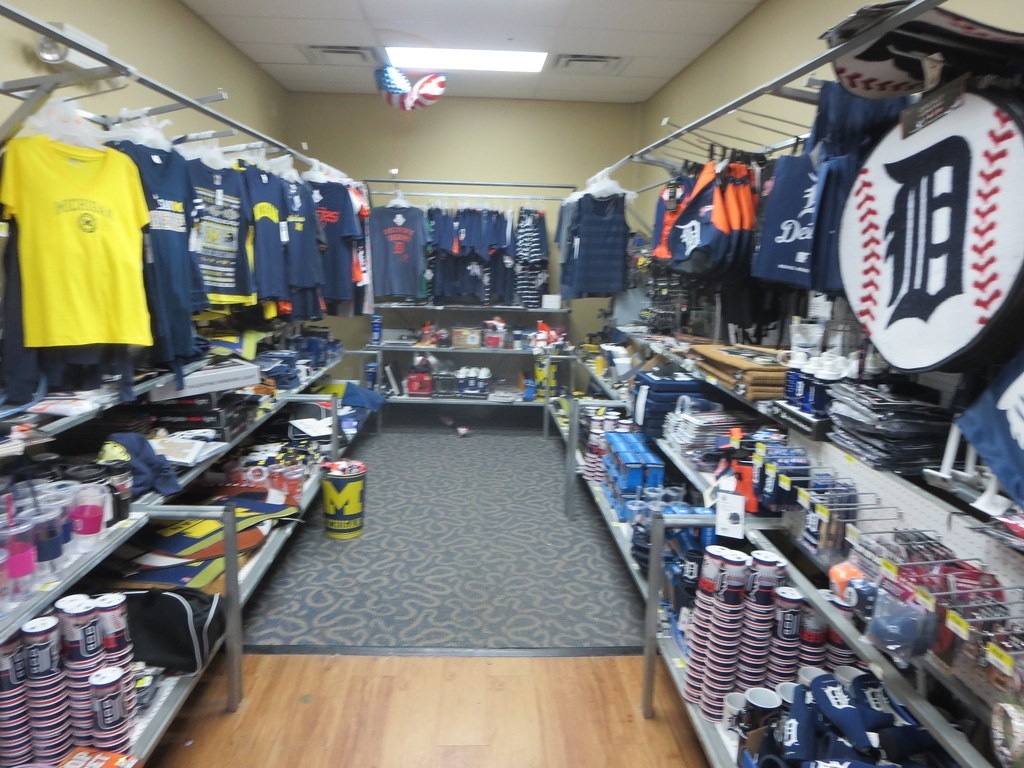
300 157 343 185
102 106 178 151
35 93 109 152
234 143 276 172
186 131 232 170
708 141 763 166
384 190 413 211
562 169 637 205
267 155 303 183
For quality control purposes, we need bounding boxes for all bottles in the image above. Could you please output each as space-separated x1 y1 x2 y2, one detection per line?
778 345 860 419
454 366 494 394
755 671 935 768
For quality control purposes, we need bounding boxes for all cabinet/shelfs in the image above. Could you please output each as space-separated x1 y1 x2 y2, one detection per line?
220 116 340 607
363 176 572 436
333 167 382 453
649 1 1024 768
551 148 648 627
1 5 238 766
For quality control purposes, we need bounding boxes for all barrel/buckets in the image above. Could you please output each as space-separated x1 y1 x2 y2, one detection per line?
482 327 508 349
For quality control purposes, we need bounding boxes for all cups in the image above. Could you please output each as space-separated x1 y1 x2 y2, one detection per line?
720 666 868 741
20 616 75 766
34 592 110 747
794 587 872 685
0 448 133 622
642 486 685 502
87 665 132 756
582 410 634 481
731 550 779 695
681 544 749 724
764 557 806 693
95 593 138 728
0 639 33 768
624 498 692 524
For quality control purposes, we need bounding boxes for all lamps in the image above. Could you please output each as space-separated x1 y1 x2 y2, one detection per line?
36 16 112 83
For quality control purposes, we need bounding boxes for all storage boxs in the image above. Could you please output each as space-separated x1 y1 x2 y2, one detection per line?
541 293 561 310
408 366 432 397
654 502 716 554
451 324 483 348
145 392 260 443
627 365 706 436
150 354 260 403
599 431 664 521
433 370 455 399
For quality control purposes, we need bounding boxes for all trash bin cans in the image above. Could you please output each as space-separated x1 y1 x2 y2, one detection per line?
320 460 368 540
